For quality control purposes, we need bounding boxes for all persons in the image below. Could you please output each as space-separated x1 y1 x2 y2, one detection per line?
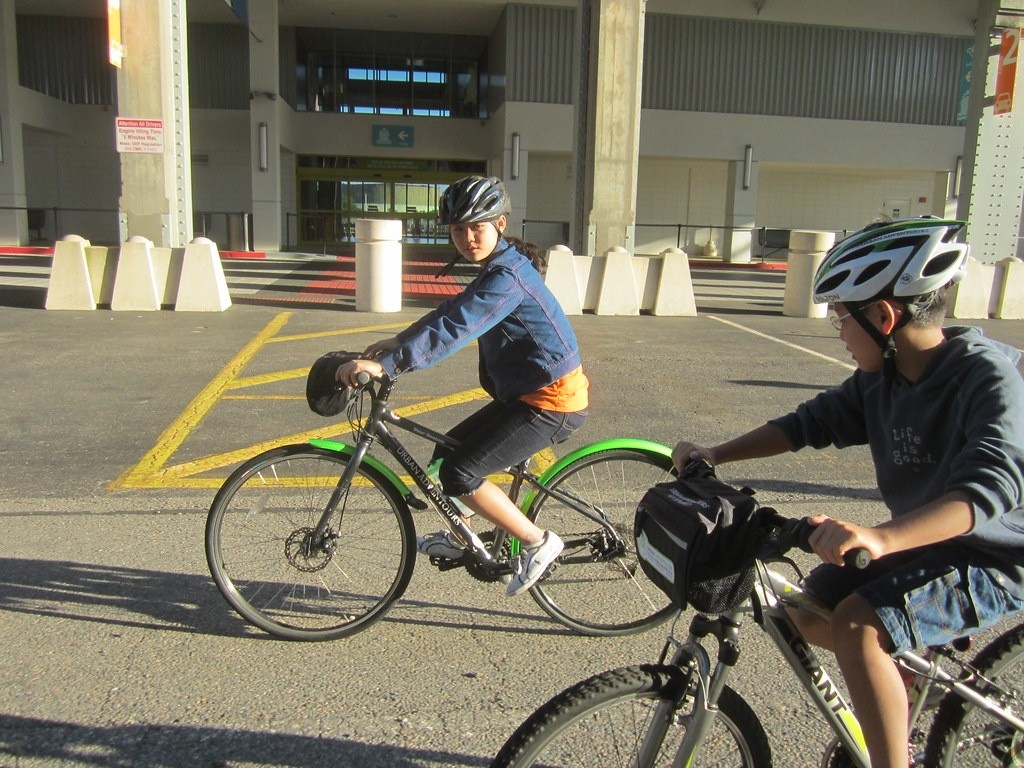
672 214 1023 768
333 175 590 597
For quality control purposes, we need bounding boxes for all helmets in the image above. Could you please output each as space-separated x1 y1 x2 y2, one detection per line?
439 176 511 222
812 214 971 305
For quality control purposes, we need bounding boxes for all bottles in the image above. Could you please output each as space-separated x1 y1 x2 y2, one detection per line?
427 457 476 517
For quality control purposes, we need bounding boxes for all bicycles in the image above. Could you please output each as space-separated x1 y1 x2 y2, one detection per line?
489 457 1024 768
204 349 733 641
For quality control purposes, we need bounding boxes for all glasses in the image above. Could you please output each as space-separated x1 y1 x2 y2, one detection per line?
830 301 903 330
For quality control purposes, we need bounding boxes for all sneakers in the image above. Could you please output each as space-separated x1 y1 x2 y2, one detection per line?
416 530 466 561
908 672 949 712
505 532 563 597
